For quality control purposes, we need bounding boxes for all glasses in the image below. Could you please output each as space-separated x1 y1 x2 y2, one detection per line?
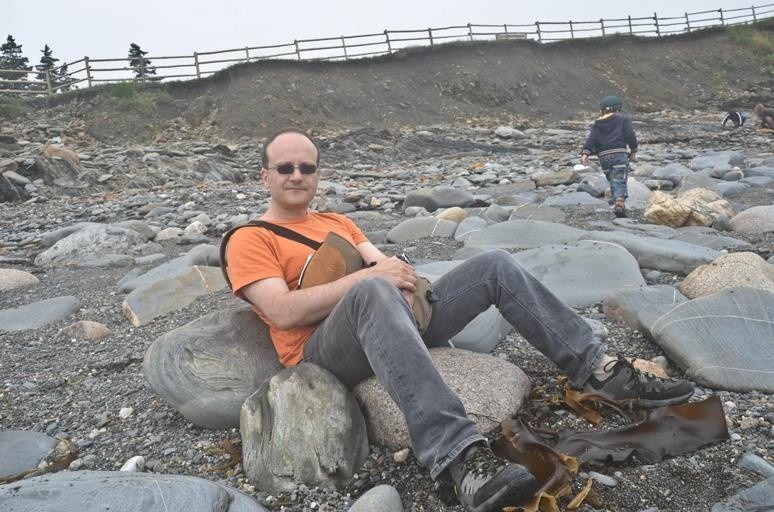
266 163 318 174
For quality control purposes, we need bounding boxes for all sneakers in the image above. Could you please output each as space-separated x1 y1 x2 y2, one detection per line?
614 199 627 218
582 355 695 409
448 444 537 512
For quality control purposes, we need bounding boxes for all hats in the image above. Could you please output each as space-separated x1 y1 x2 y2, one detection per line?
599 95 623 109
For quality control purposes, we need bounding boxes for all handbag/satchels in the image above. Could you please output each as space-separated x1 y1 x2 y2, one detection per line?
295 230 436 336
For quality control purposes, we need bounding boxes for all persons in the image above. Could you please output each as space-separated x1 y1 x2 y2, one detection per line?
755 104 774 128
582 95 638 218
720 109 745 128
225 128 695 512
